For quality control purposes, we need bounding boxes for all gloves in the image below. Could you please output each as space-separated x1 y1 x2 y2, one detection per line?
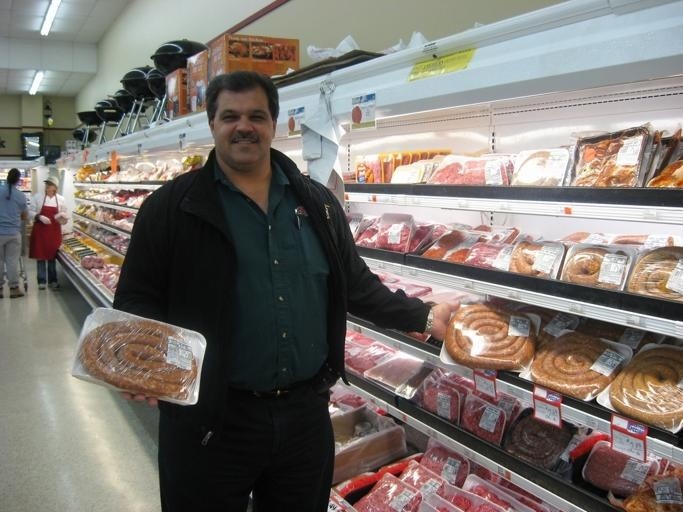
39 216 51 224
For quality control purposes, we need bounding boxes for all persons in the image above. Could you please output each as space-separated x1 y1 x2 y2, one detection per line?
111 69 461 512
0 167 27 299
28 175 67 290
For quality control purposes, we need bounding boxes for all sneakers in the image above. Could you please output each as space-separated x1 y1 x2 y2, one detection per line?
48 282 60 288
39 283 46 290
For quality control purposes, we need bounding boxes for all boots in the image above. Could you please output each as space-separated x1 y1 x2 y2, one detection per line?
10 286 24 298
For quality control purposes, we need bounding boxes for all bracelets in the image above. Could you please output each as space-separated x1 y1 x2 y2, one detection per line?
426 308 437 334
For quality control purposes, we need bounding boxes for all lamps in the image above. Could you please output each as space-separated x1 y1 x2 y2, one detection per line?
42 96 53 118
37 1 63 38
26 70 48 96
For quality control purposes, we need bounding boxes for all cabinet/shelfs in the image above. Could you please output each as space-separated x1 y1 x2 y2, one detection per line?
0 177 34 224
335 178 683 511
56 160 181 306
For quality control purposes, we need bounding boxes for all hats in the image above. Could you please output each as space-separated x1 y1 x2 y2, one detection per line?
44 176 58 187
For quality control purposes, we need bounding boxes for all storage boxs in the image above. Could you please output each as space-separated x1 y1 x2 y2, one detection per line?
326 407 411 478
166 32 298 117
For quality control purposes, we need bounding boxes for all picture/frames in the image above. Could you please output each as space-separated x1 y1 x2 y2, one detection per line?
20 131 43 162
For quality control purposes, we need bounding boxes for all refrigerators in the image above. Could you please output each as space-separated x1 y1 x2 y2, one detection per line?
0 159 37 231
46 0 682 512
